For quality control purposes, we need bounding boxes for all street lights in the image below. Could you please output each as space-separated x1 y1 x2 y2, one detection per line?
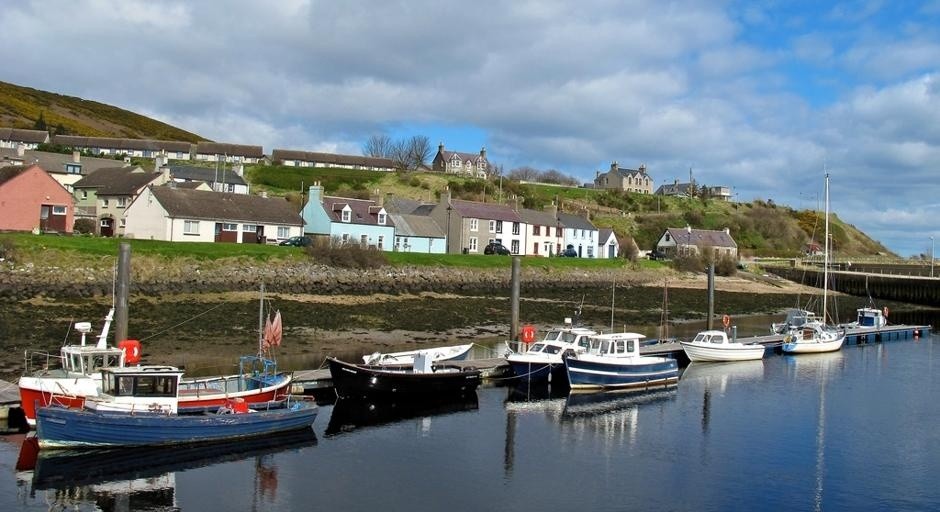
927 235 937 260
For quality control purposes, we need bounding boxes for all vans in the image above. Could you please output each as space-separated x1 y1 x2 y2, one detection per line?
559 249 577 257
484 243 510 255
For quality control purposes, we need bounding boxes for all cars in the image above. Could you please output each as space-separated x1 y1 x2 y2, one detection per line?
279 236 315 247
647 249 666 261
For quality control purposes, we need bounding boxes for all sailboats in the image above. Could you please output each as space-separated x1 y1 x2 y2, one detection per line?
770 173 845 354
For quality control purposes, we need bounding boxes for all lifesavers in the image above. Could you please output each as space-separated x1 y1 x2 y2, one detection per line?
722 315 730 325
884 306 889 316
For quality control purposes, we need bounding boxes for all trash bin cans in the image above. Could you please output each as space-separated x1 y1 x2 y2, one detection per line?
258 236 266 244
464 248 469 255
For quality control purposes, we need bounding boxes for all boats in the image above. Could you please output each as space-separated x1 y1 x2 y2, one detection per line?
838 303 889 330
